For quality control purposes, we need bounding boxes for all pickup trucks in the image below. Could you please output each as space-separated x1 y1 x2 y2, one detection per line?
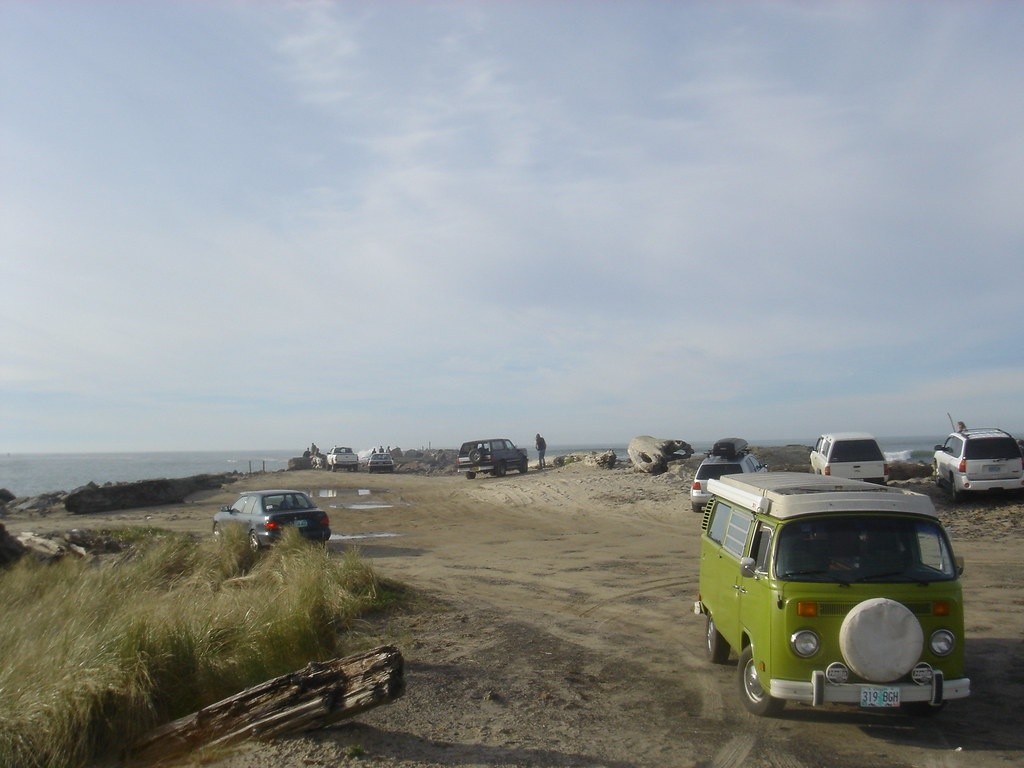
326 447 358 472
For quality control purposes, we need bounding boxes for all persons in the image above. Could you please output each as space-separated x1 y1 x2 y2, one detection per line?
536 434 547 469
303 443 319 456
372 446 391 455
957 422 967 431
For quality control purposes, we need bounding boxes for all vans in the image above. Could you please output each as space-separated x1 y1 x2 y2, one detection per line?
694 470 972 717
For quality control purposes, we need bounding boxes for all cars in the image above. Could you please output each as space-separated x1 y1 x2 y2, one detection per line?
212 490 331 553
368 453 394 473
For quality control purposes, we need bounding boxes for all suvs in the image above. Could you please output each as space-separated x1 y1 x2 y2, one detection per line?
457 439 528 479
690 438 768 512
933 428 1024 502
807 433 888 487
